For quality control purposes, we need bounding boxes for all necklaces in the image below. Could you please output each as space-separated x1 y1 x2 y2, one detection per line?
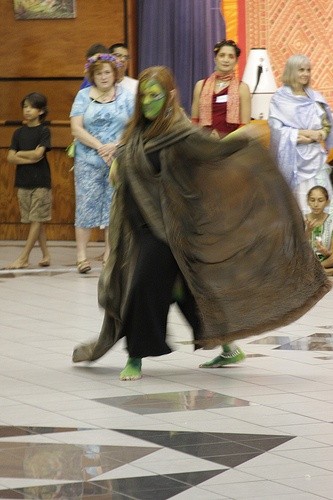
215 82 229 87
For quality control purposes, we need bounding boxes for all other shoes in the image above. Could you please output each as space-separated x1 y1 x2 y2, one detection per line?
76 259 91 274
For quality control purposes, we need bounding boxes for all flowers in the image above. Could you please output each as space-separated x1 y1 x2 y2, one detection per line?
83 54 124 71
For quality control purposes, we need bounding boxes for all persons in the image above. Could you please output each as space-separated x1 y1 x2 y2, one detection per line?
70 43 138 273
268 54 333 214
73 66 333 380
303 186 333 275
7 92 52 268
191 39 251 140
